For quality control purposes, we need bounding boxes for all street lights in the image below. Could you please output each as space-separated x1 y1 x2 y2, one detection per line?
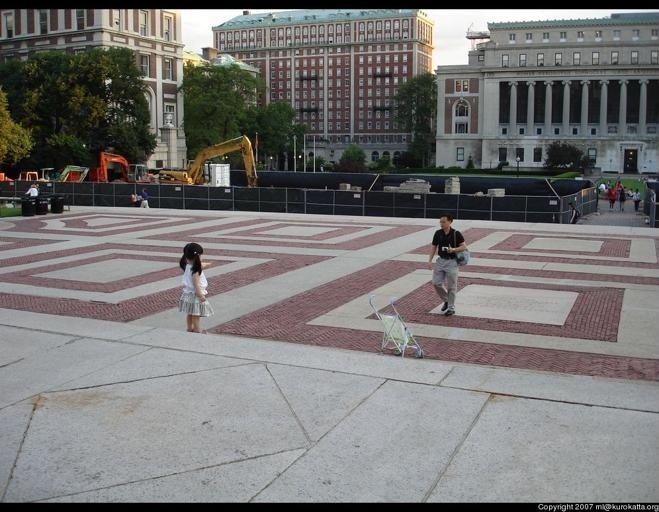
224 153 230 164
270 153 274 172
515 155 522 178
297 154 305 173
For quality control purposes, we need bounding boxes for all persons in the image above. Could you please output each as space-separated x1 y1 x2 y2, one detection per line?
427 213 468 316
597 180 642 212
25 183 41 199
568 202 580 223
178 243 215 332
140 188 150 208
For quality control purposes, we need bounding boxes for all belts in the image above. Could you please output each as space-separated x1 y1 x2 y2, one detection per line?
441 257 454 260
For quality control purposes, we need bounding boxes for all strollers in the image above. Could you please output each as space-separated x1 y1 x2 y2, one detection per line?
369 291 425 362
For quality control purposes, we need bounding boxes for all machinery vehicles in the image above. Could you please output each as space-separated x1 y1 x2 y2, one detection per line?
156 133 261 190
0 163 93 188
88 149 159 187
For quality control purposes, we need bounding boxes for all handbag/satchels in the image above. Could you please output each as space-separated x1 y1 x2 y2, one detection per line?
454 229 470 267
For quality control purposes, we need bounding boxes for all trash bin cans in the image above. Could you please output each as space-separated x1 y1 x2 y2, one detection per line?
21 194 65 216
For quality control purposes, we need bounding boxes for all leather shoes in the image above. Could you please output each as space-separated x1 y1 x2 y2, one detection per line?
441 302 448 311
445 310 455 316
